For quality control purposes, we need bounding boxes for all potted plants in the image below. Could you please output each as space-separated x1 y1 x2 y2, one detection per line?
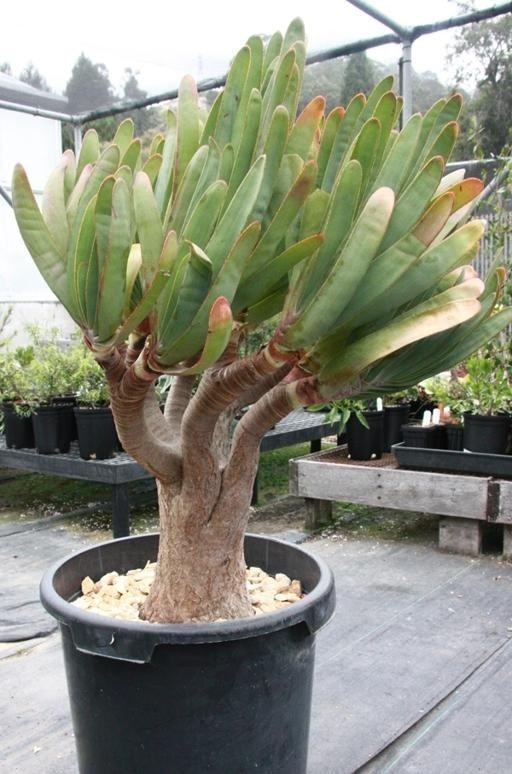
1 325 126 460
305 357 512 460
12 18 512 774
